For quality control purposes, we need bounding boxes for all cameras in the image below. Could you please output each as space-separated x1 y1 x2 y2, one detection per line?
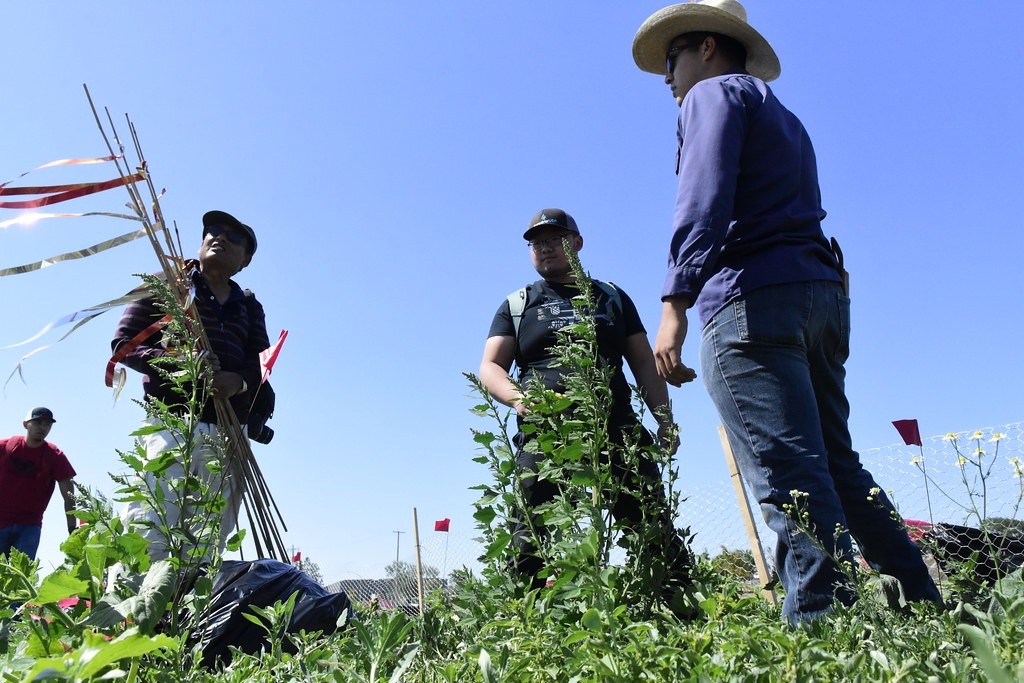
248 423 274 445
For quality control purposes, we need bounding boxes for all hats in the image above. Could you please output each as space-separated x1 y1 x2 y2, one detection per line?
26 407 57 423
520 209 581 234
203 208 259 250
630 0 781 82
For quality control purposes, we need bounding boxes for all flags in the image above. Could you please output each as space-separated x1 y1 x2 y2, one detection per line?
435 518 450 532
891 419 923 447
258 329 289 385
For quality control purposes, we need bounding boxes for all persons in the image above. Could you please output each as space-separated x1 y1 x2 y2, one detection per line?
480 208 699 625
0 407 78 626
632 0 946 637
103 211 276 601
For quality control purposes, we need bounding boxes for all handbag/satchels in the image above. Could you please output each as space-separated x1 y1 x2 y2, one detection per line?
170 557 354 670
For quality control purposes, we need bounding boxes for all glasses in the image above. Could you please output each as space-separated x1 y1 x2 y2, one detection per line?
205 221 247 247
527 234 568 255
663 43 694 72
